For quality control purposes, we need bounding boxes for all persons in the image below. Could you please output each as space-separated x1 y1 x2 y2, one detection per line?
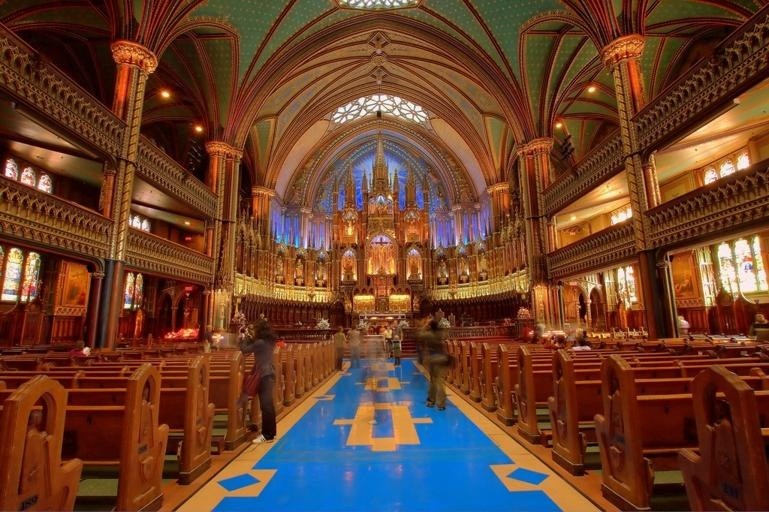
513 320 593 353
392 320 403 343
421 313 458 410
391 334 403 365
384 324 394 359
68 339 87 360
749 312 768 337
333 326 347 372
276 336 288 349
346 323 362 369
240 317 279 445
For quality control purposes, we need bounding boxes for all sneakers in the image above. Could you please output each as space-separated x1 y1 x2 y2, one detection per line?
252 435 274 444
426 399 435 407
437 407 446 410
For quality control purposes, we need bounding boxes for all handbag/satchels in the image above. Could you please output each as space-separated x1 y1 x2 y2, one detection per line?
243 373 262 397
